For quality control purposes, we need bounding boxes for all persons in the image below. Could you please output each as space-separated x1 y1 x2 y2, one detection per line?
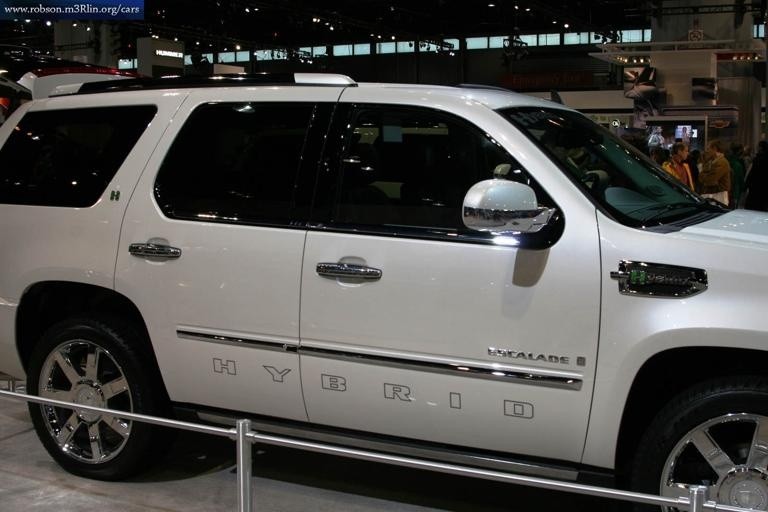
683 149 701 195
725 142 747 209
698 139 731 207
743 139 768 212
662 141 695 193
647 126 665 149
680 127 690 145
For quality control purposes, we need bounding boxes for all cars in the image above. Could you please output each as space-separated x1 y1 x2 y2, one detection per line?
0 72 767 512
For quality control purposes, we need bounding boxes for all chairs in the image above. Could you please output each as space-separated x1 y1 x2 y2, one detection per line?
340 164 465 226
